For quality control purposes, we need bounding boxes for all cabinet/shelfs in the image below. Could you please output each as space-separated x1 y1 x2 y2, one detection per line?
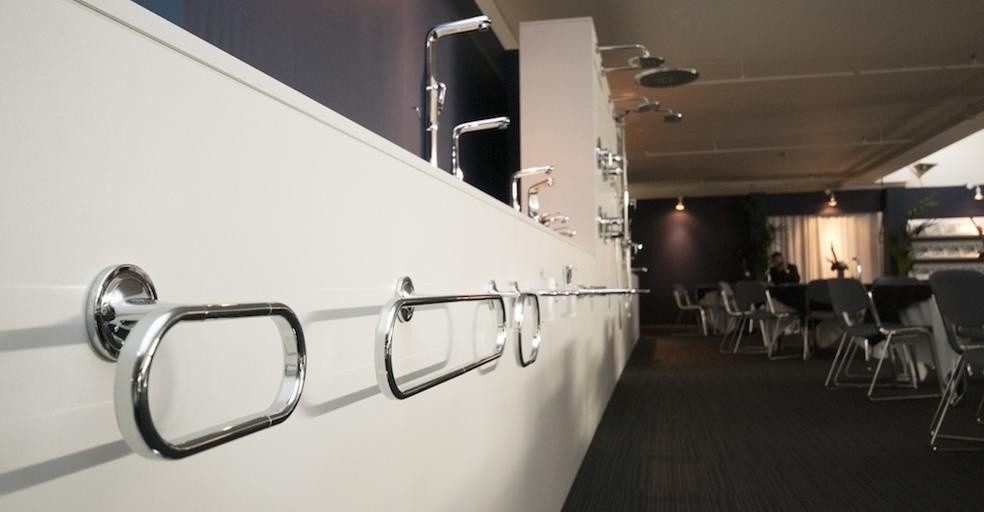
906 215 984 284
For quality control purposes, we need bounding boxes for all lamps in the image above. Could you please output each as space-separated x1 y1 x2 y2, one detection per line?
828 192 838 207
974 184 983 201
675 195 685 211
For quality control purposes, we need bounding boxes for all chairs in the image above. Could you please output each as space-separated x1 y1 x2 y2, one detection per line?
671 269 984 455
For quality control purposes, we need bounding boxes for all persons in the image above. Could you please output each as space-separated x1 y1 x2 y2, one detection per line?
769 250 800 283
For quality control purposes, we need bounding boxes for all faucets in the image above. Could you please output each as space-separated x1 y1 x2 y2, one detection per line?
558 229 577 240
508 164 554 212
411 14 491 167
528 177 554 222
451 116 512 180
540 211 570 227
